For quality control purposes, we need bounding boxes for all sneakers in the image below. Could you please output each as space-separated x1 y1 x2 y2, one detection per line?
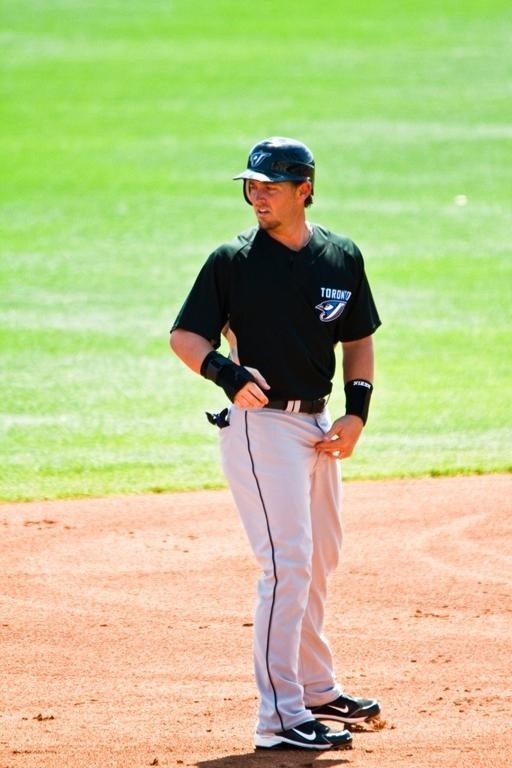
256 694 381 751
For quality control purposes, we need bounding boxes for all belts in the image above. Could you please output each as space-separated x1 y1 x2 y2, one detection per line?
263 398 326 414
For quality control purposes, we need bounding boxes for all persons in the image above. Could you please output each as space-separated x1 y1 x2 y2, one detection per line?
169 136 380 749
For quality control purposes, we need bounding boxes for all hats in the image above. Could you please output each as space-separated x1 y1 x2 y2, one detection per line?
232 137 315 183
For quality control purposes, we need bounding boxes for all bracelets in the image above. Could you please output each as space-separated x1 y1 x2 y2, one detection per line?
345 380 373 426
